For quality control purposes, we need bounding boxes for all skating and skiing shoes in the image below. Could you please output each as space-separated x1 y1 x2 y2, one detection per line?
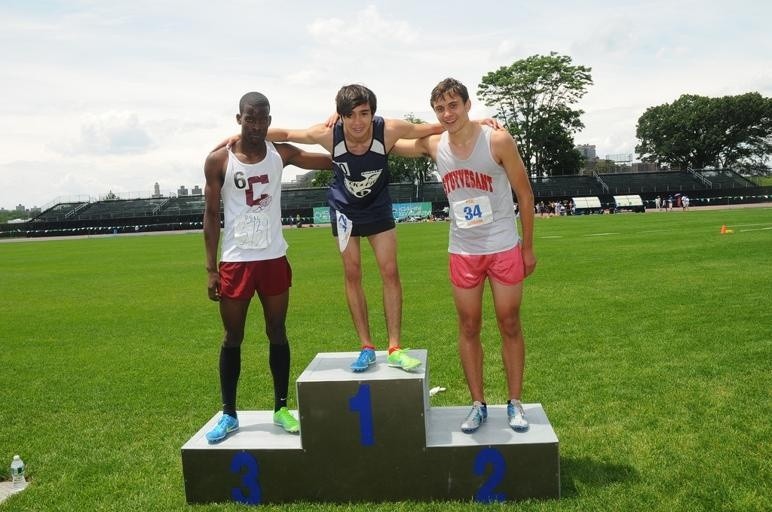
386 348 422 372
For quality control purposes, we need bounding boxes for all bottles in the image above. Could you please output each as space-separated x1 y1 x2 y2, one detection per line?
9 454 28 494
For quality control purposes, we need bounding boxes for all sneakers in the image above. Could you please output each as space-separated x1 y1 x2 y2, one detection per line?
272 406 300 433
349 347 377 373
206 413 240 442
460 400 488 433
506 398 529 432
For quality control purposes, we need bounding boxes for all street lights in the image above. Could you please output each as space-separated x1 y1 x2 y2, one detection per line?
413 177 420 198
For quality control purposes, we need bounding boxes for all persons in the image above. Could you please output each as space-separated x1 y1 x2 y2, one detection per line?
204 92 337 443
326 79 536 432
208 85 504 371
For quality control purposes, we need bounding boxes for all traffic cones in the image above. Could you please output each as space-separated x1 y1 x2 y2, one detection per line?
719 223 728 234
